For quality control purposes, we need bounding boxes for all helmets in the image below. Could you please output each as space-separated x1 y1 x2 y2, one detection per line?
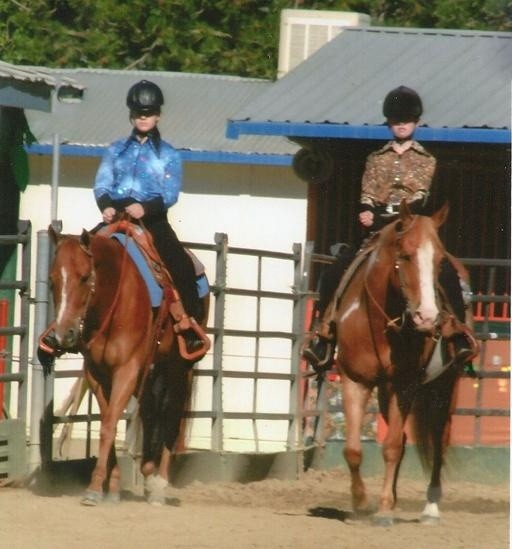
125 79 165 113
381 84 423 121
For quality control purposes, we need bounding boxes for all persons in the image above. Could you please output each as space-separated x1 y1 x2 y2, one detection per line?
45 79 205 354
303 86 476 371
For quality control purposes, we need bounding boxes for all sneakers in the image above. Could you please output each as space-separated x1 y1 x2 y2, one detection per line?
181 331 207 361
451 333 473 360
42 326 61 353
301 336 329 363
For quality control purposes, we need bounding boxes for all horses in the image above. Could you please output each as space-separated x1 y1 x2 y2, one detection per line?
45 221 211 506
329 196 474 529
